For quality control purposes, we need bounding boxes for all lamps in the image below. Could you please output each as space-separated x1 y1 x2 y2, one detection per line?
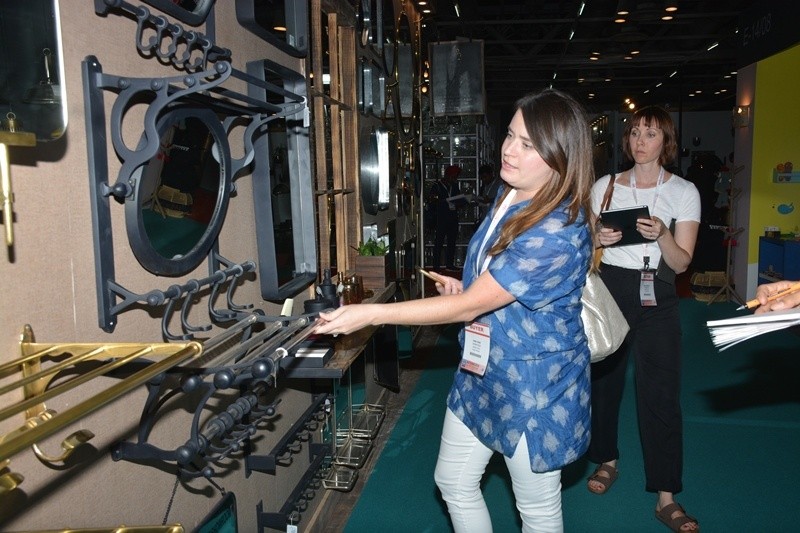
732 103 749 128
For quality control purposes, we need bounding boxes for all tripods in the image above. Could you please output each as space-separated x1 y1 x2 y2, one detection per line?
706 165 746 311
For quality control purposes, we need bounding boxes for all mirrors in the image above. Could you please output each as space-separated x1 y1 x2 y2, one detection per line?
136 108 232 263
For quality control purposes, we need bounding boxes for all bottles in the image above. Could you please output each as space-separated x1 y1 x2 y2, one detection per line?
764 226 781 240
303 268 363 339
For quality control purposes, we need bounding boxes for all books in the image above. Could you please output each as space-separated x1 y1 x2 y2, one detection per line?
707 308 800 353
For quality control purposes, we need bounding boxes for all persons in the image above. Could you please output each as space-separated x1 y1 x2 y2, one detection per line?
587 103 701 533
313 87 591 533
755 281 800 314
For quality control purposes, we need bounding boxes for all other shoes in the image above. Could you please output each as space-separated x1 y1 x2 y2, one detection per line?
445 265 461 272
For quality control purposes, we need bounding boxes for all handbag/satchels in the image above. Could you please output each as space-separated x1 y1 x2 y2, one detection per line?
580 270 630 364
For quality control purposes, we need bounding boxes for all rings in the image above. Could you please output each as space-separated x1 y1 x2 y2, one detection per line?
333 333 338 337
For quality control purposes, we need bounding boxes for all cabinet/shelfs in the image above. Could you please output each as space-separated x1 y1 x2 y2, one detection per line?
758 235 800 284
415 119 499 298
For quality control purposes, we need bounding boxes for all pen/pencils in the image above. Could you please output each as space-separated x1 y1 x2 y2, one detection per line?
736 283 800 311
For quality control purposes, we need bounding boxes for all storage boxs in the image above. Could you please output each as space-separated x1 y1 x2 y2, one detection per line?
691 272 730 303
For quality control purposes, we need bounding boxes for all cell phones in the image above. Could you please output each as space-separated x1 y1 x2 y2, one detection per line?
414 263 446 287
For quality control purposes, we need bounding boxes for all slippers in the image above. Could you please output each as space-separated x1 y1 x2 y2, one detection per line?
587 463 618 494
656 502 699 533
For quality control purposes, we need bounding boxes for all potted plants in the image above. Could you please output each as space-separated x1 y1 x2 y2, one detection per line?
354 237 393 289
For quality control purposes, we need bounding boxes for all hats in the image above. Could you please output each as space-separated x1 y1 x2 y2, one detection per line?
445 165 463 176
